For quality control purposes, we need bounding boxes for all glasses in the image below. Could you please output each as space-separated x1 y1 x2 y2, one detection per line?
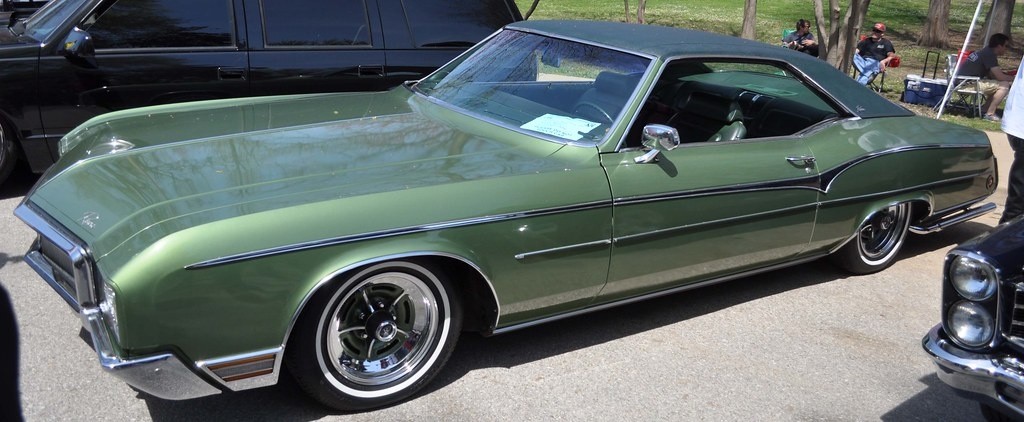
804 26 810 28
872 29 881 33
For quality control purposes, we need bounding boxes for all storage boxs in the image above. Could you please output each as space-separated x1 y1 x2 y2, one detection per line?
886 56 900 67
903 50 951 107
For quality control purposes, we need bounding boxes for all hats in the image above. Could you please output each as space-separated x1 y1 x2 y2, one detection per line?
871 23 886 31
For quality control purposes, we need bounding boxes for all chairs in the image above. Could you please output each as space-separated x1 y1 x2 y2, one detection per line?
851 35 891 93
933 53 987 122
660 90 747 146
571 71 639 127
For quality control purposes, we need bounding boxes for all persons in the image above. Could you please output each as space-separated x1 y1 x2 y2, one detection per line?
954 33 1015 121
781 19 819 58
853 22 895 86
998 54 1024 226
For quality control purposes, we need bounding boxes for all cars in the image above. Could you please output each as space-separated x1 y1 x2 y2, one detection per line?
18 20 1004 417
921 214 1024 422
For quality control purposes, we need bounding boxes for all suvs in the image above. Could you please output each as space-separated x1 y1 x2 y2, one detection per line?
0 0 539 199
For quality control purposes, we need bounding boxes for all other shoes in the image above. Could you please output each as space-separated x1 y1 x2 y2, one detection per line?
982 111 1001 121
867 72 877 84
